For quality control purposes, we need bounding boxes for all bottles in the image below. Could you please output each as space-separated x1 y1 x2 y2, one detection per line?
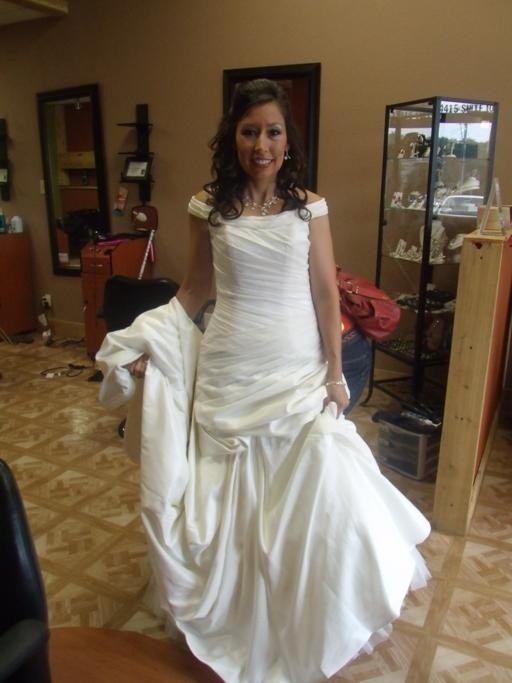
1 208 24 233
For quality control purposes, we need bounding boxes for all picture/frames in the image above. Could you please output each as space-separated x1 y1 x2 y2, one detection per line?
121 155 154 183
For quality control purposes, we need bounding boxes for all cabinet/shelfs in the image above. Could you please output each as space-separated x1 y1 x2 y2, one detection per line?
0 232 41 343
81 236 152 359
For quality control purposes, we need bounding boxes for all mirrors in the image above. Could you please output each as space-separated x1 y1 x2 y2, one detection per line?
221 62 321 194
37 83 110 277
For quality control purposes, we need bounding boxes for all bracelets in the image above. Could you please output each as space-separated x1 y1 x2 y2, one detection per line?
325 380 346 385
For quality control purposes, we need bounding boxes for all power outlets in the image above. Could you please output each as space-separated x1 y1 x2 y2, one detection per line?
41 293 51 309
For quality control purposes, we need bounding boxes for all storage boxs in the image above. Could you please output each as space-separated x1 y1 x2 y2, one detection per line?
375 417 441 481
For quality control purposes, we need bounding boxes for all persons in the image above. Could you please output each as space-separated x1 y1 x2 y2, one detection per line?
94 78 434 683
338 304 375 415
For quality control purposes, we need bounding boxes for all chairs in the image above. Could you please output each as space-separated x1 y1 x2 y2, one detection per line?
96 273 216 437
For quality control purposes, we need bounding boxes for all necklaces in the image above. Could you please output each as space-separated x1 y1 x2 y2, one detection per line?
242 192 279 215
